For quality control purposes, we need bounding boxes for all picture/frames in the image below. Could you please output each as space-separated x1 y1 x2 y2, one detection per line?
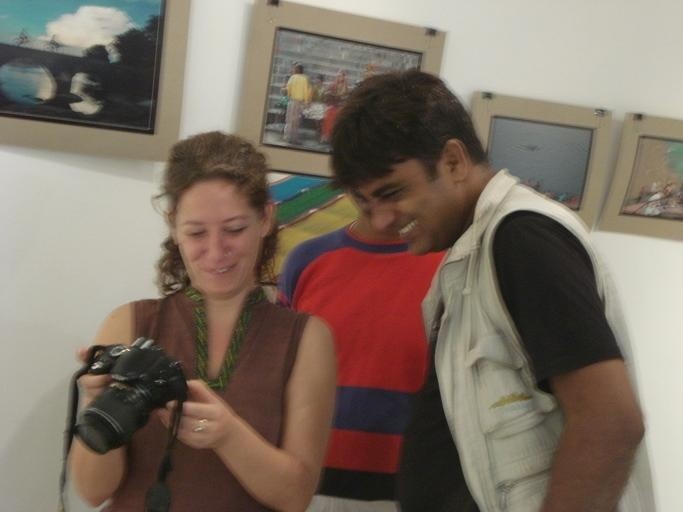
235 0 448 179
1 1 191 163
598 113 681 242
469 91 616 232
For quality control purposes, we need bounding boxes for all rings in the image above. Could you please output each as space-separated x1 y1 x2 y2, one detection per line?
192 418 210 432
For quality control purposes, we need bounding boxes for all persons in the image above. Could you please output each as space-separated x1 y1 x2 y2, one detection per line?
322 69 656 512
281 61 312 145
62 129 340 512
316 69 351 146
270 207 449 512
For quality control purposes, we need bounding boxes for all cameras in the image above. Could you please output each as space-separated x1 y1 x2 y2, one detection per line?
74 337 189 455
